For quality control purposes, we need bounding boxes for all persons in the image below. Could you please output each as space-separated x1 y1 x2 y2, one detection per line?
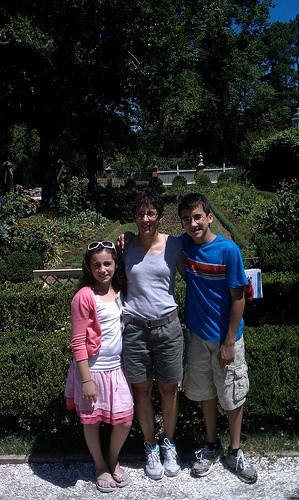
115 193 258 483
64 241 134 493
112 194 183 479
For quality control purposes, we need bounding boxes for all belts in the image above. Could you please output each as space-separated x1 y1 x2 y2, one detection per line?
132 309 178 329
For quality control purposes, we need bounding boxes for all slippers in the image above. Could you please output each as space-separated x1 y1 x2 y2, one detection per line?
95 476 117 493
112 468 131 488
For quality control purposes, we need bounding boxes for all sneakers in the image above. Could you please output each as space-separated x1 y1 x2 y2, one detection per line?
144 441 164 480
191 438 224 476
226 445 257 484
160 438 182 477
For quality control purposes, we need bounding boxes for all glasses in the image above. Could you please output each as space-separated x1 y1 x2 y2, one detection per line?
135 211 158 220
182 214 207 224
88 241 117 256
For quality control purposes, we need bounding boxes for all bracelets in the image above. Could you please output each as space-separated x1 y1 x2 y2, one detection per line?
81 379 93 383
